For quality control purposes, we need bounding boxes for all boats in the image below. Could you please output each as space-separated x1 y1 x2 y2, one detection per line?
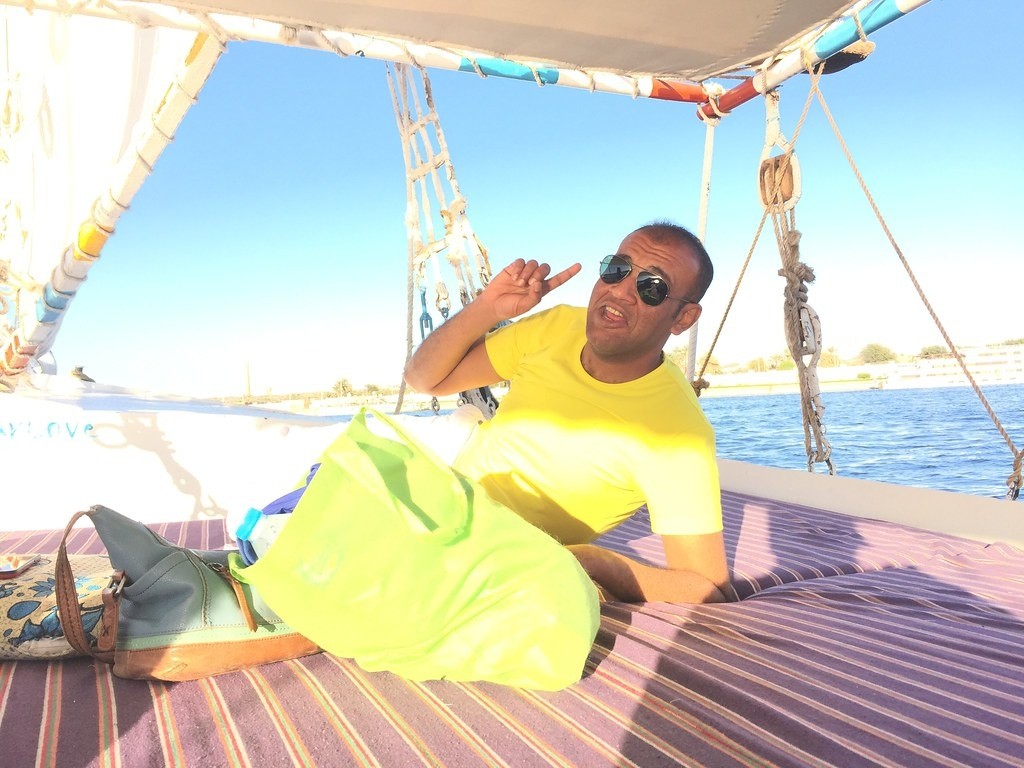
882 346 1024 389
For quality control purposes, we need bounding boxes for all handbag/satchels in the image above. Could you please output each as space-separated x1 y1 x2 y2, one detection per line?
55 505 325 682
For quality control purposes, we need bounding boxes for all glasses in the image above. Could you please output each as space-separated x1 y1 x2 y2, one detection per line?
599 255 690 306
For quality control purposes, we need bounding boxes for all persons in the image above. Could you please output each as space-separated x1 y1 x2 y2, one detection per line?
403 223 732 604
